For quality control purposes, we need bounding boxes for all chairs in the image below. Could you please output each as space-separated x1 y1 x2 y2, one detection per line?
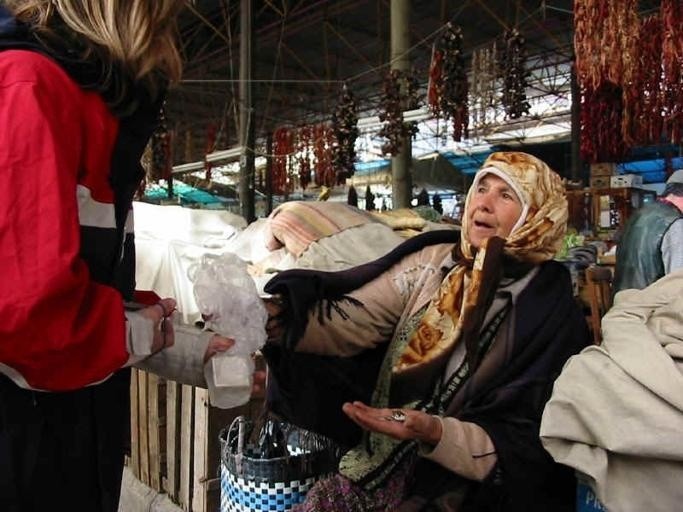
585 268 613 345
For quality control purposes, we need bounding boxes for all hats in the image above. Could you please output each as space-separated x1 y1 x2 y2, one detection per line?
665 169 683 185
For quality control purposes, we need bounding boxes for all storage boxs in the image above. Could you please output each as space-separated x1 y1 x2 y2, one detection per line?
588 162 643 190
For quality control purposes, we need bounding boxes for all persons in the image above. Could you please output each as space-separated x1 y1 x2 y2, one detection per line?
0 1 266 510
198 150 593 512
606 167 683 312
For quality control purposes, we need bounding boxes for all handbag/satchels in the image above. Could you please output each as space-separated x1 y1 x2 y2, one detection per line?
217 417 341 512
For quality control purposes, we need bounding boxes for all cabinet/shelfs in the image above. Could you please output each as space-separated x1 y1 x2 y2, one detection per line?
594 187 656 233
